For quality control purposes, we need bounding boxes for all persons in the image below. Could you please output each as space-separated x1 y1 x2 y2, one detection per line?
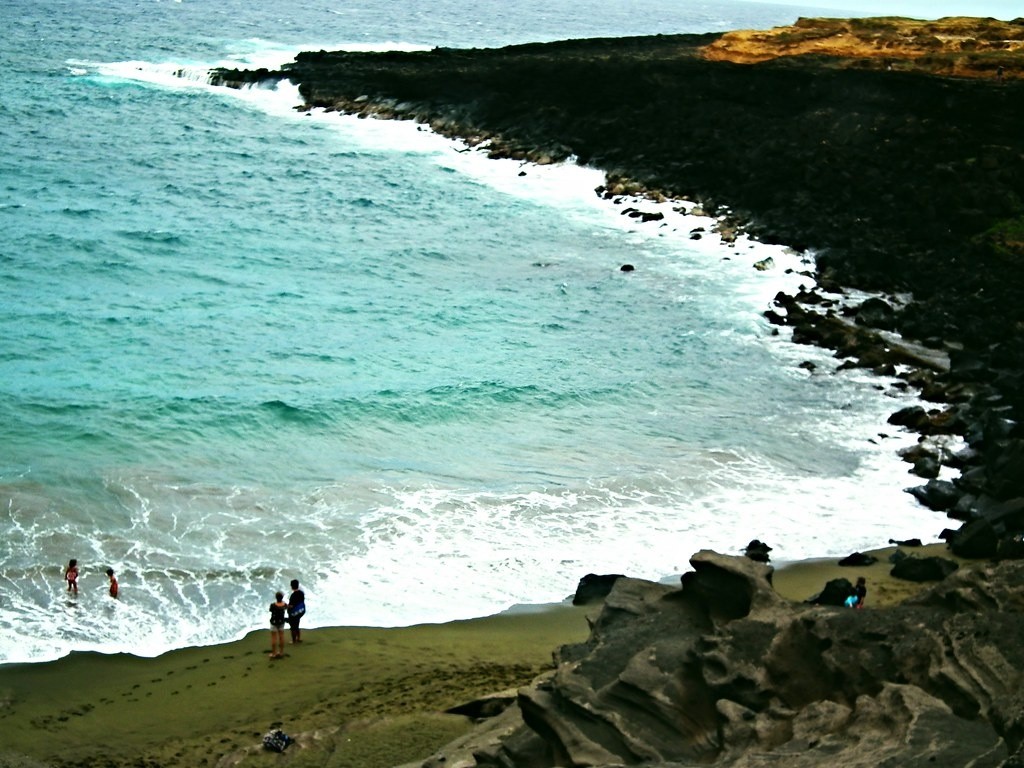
844 588 859 609
269 592 288 658
853 576 868 608
287 579 306 644
64 558 79 594
106 569 118 600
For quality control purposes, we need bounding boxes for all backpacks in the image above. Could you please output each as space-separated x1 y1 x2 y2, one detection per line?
263 729 295 753
